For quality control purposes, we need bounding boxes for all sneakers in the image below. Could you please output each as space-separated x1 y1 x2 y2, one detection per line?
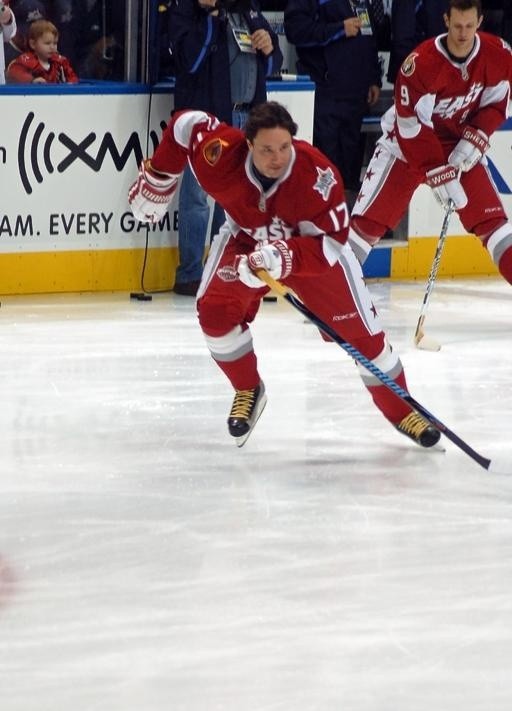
395 411 440 447
173 281 200 296
227 379 265 437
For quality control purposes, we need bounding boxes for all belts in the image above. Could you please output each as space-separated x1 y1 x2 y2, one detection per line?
234 102 251 112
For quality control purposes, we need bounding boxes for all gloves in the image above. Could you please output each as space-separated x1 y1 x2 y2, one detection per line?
129 160 183 224
234 240 292 289
448 126 489 173
426 164 468 210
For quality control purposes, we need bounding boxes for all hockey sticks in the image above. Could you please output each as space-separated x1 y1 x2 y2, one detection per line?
415 163 464 353
254 269 512 475
